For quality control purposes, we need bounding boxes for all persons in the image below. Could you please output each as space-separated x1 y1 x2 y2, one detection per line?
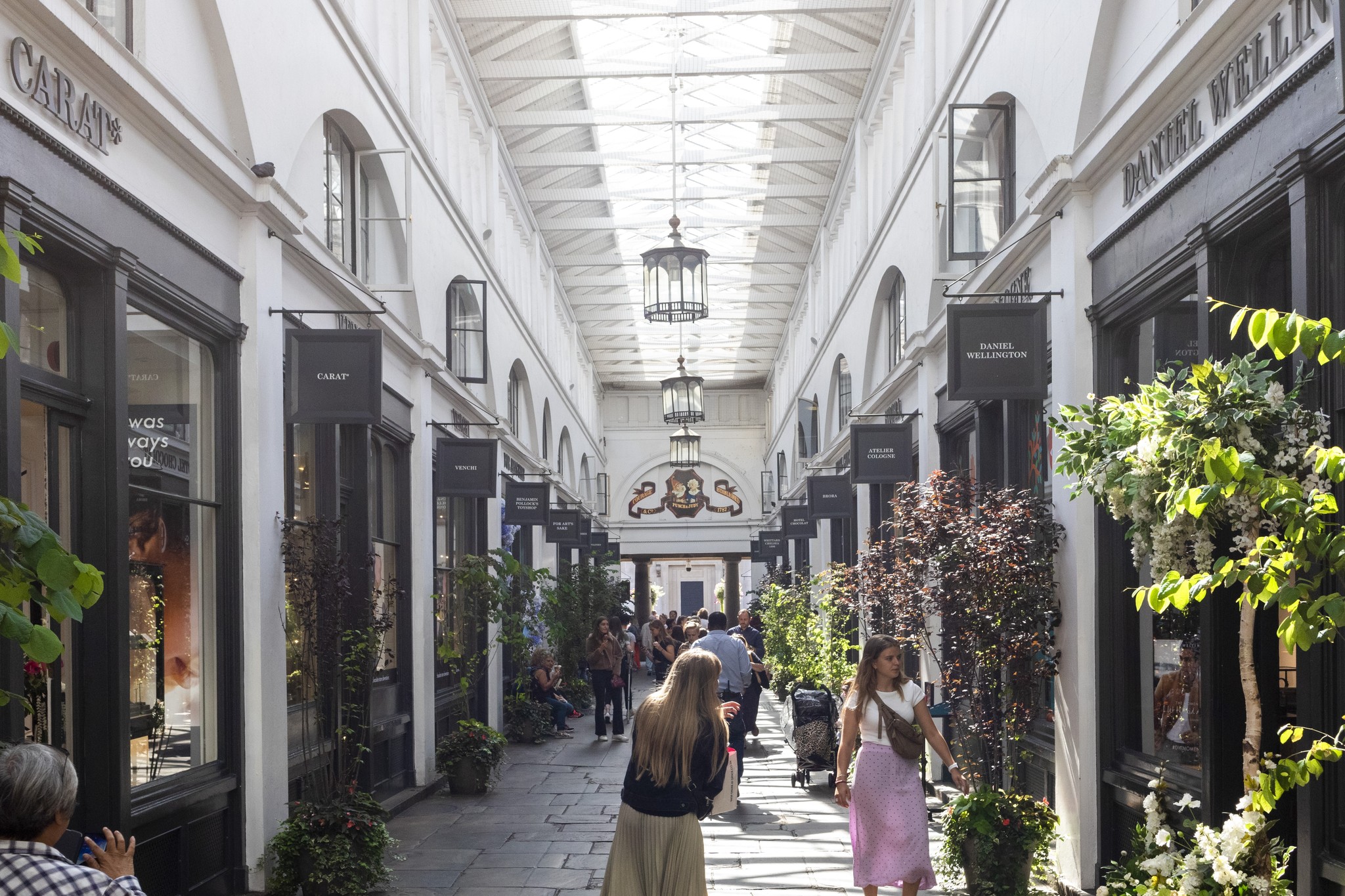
691 611 752 798
1154 637 1204 765
640 607 765 750
529 647 585 738
596 647 741 896
129 501 199 697
586 616 629 742
834 634 969 896
0 743 146 896
586 617 641 723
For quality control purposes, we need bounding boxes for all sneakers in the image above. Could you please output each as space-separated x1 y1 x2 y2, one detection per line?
544 727 558 736
567 710 585 720
611 734 629 742
555 730 574 739
564 723 575 732
598 735 608 741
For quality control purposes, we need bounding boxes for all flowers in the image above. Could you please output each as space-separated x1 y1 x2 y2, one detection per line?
819 296 1345 896
430 548 589 795
630 583 667 609
246 513 410 896
714 578 744 606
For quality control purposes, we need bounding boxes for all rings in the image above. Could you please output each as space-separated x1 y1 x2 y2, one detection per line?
836 798 838 802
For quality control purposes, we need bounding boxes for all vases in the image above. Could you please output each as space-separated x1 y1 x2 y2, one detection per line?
298 855 367 896
961 836 1034 892
445 757 488 795
519 714 535 744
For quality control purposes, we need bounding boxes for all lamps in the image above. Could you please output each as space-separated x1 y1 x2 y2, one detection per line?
658 326 706 425
669 424 702 468
640 14 709 323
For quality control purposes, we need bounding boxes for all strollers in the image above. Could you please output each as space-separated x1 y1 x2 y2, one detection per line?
779 683 841 788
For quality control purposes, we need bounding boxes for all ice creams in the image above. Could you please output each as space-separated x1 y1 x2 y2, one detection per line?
554 665 562 672
604 634 608 642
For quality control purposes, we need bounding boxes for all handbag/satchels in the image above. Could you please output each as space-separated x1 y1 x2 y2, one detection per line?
607 677 626 693
866 680 925 759
706 708 739 816
749 650 770 689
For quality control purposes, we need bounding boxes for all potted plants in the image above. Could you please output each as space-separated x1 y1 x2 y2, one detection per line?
761 585 815 704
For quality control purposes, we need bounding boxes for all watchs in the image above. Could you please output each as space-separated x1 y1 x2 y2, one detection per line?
948 762 961 773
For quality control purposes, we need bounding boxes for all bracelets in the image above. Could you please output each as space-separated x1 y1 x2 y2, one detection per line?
836 774 847 780
835 780 847 785
660 648 663 652
645 652 649 655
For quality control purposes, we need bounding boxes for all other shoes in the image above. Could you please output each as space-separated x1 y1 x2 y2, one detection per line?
626 707 638 718
751 723 760 736
605 712 612 724
647 671 652 676
